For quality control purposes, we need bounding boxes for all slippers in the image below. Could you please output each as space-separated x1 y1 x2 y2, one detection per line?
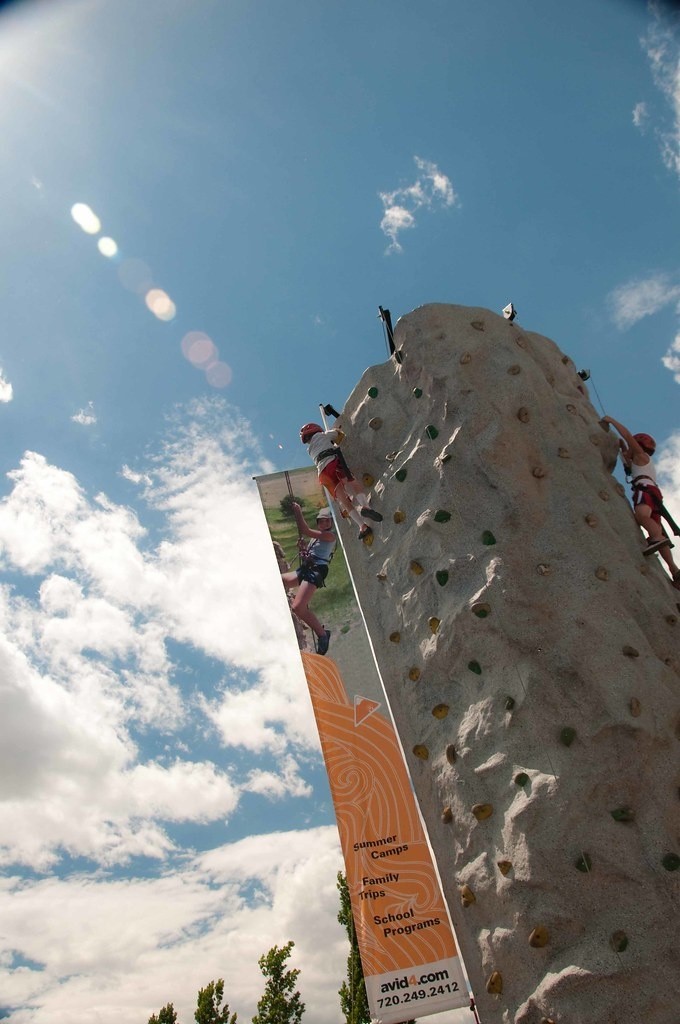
672 569 680 590
642 536 671 556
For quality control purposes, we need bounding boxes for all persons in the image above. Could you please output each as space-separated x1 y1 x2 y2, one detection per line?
299 422 383 539
280 501 338 657
601 415 680 591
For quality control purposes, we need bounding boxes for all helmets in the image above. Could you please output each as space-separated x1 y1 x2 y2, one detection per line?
316 507 332 519
299 423 324 444
632 433 657 457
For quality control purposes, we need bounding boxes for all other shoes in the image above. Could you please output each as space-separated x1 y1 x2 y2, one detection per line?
360 506 383 523
357 524 374 540
317 629 331 656
